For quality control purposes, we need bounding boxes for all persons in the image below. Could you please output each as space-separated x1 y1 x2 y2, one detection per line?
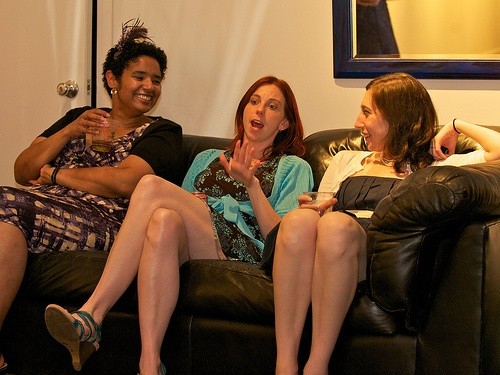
272 72 500 375
0 14 187 371
45 75 313 375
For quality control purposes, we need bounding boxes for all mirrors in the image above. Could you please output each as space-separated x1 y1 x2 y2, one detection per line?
332 0 500 79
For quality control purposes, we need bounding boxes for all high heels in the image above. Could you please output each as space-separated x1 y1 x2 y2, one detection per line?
44 304 102 371
137 362 167 375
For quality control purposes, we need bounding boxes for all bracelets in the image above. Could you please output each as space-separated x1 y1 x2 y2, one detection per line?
453 118 461 134
51 168 60 187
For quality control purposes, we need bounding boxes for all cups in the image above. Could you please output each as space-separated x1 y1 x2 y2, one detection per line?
91 120 119 152
303 192 336 216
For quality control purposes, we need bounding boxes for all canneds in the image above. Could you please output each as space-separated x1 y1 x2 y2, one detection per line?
190 192 208 205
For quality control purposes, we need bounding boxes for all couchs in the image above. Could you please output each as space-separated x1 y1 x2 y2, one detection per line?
0 125 500 374
